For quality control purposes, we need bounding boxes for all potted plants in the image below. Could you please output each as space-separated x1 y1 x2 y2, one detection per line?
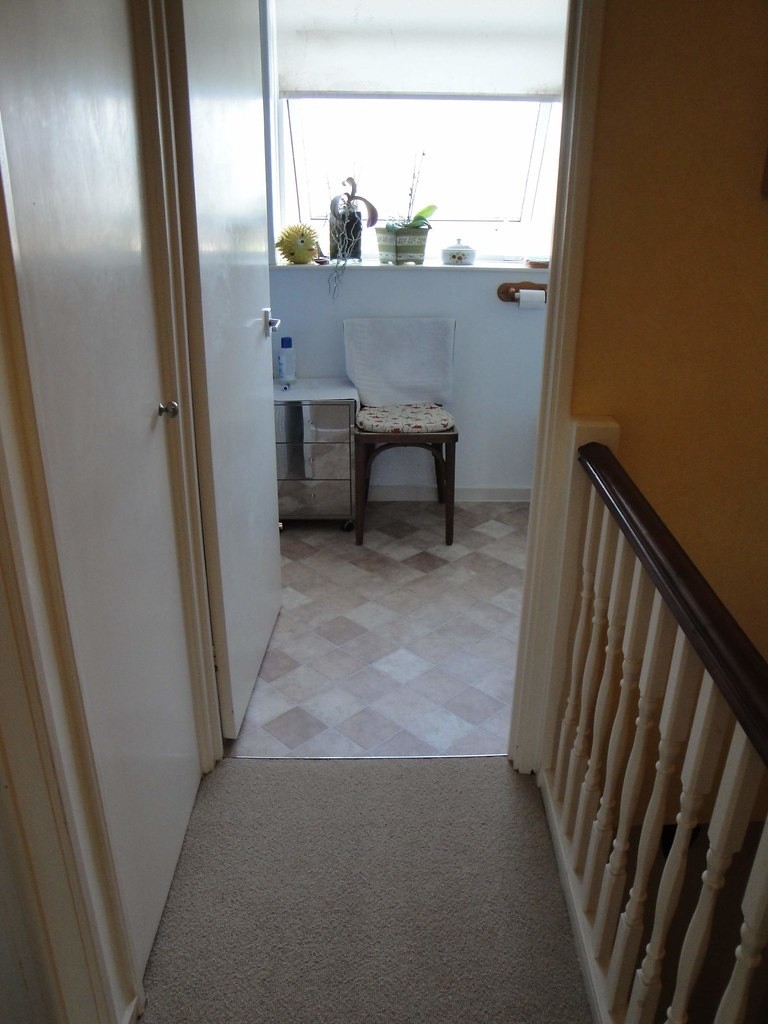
328 176 377 263
372 151 433 264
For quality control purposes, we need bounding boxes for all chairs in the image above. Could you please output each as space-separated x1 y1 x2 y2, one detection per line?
344 316 457 545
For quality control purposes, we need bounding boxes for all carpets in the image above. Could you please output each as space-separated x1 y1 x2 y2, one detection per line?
127 760 597 1024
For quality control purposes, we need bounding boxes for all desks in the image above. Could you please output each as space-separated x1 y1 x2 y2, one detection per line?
273 378 355 531
